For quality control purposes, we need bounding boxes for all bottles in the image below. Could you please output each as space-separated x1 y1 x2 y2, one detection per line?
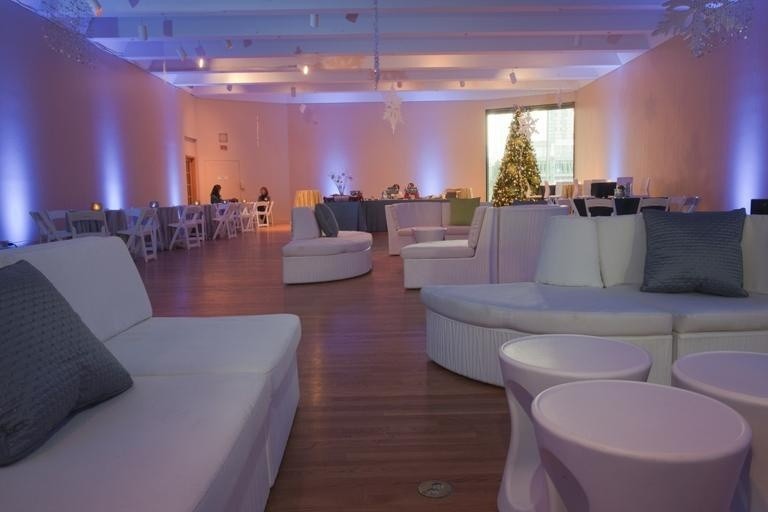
614 183 630 197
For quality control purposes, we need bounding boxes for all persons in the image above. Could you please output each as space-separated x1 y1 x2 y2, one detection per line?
391 184 403 199
404 183 419 199
257 186 271 224
210 184 227 204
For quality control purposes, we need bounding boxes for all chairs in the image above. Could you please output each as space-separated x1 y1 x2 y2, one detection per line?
554 176 699 216
29 202 273 262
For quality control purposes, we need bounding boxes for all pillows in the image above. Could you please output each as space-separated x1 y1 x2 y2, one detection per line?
0 260 133 467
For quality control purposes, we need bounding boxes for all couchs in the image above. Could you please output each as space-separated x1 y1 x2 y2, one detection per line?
0 235 303 512
